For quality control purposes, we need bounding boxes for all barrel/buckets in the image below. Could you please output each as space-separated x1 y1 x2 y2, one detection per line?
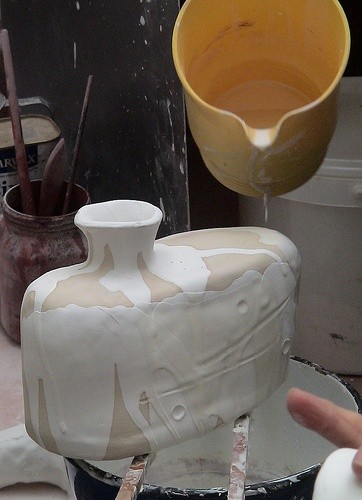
231 70 360 384
0 139 50 201
0 178 92 350
65 353 360 499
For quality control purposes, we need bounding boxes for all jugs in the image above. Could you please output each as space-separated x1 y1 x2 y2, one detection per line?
171 0 351 197
